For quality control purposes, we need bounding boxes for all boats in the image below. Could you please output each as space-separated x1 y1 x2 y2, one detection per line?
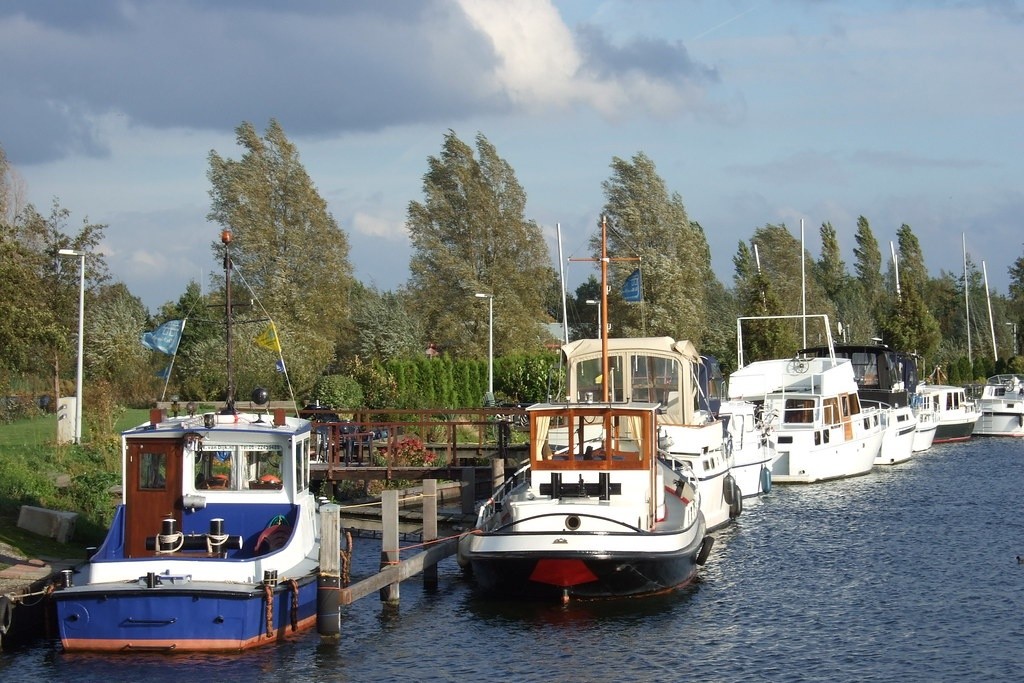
535 219 1024 533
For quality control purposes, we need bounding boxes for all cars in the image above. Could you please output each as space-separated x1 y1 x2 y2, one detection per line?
293 405 351 452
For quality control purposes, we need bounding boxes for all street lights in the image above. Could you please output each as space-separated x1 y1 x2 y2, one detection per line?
58 249 86 444
585 301 601 373
475 293 494 395
1005 323 1017 356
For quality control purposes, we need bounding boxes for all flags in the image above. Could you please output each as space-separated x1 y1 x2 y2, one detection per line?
141 318 184 355
620 268 641 302
154 365 171 378
275 359 286 373
254 322 282 352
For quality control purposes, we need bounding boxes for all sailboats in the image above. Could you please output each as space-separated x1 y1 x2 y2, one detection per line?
467 219 706 607
51 231 353 651
